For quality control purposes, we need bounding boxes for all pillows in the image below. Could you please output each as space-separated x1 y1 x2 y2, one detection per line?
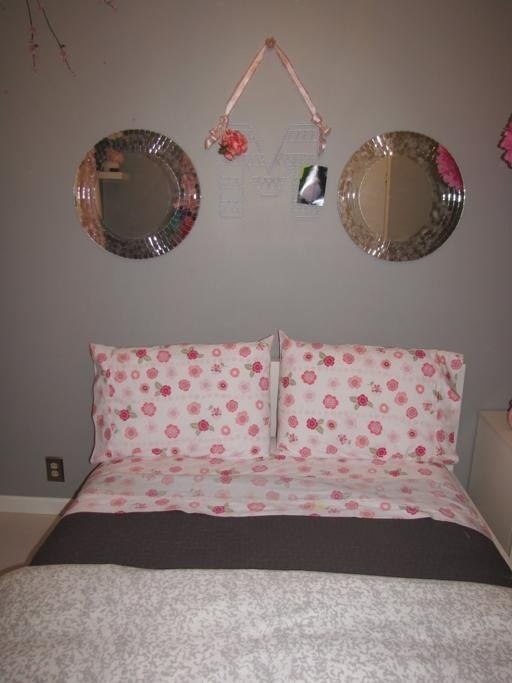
85 335 274 468
276 329 465 473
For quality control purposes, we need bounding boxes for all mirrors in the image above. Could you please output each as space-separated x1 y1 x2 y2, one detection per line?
335 130 466 265
72 125 201 261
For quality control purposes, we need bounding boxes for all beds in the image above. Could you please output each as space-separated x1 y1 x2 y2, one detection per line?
15 361 511 683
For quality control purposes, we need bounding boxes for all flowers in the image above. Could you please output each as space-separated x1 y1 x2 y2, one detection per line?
217 127 248 162
